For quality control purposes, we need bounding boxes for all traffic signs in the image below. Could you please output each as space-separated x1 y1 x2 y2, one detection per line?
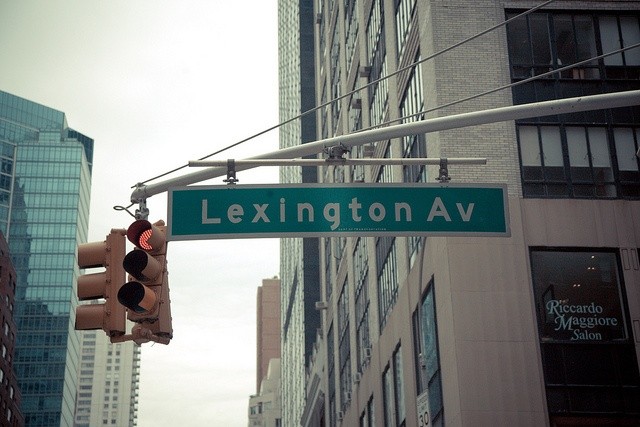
168 182 511 236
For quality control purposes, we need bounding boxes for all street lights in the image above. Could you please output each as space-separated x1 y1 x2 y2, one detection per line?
74 227 126 342
117 218 172 345
